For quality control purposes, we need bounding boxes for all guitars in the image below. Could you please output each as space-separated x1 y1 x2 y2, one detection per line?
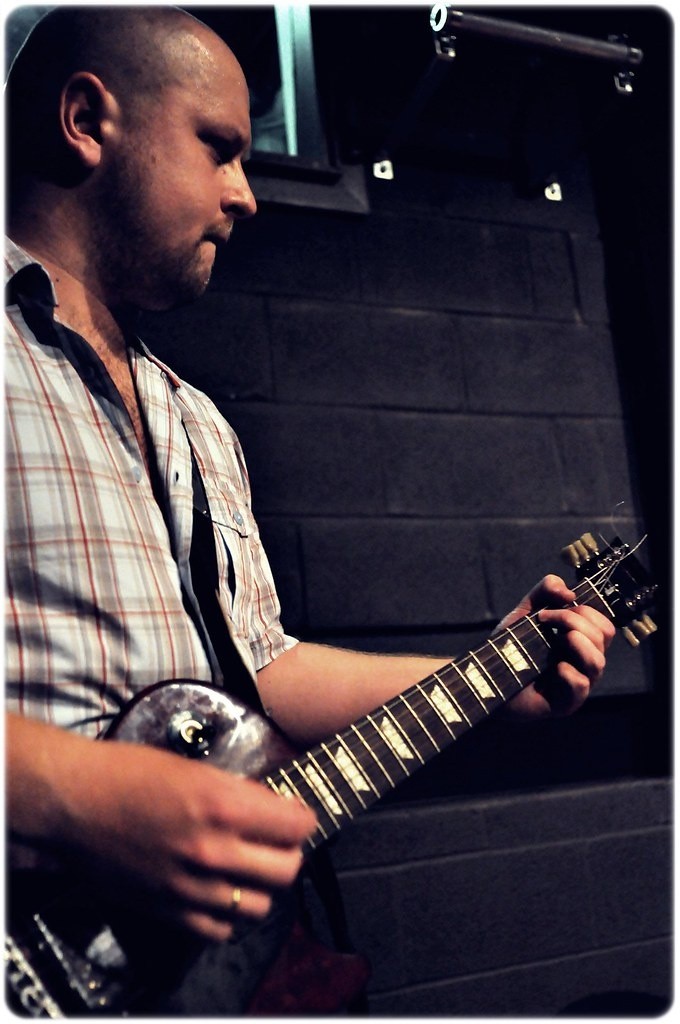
4 532 673 1018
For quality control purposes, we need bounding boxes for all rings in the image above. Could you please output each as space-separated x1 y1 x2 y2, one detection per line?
231 886 240 911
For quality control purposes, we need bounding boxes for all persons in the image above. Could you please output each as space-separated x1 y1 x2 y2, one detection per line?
5 4 616 1020
